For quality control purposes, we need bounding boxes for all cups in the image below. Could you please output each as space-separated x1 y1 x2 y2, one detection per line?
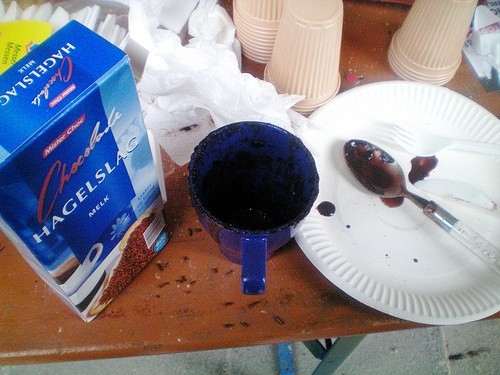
388 0 478 86
232 0 284 64
188 121 319 295
263 0 344 117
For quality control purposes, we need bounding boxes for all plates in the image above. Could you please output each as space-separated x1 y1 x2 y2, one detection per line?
294 80 500 324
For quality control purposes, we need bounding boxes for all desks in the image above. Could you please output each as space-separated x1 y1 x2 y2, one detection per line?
1 0 499 375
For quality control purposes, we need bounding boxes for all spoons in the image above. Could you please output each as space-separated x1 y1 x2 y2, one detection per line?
344 139 499 274
